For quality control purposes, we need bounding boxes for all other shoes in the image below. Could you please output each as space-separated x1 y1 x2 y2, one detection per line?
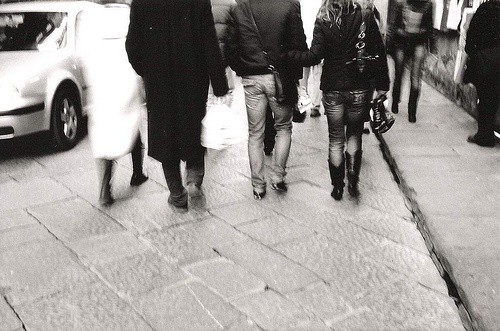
468 134 497 146
98 190 114 204
253 191 266 199
272 182 287 192
310 109 320 117
168 195 188 209
131 173 148 186
188 184 204 199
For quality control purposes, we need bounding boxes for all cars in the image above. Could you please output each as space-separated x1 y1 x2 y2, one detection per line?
0 0 107 152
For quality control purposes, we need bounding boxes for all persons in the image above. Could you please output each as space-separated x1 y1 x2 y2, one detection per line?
124 0 229 208
461 0 500 148
74 0 150 208
205 0 390 202
384 0 436 124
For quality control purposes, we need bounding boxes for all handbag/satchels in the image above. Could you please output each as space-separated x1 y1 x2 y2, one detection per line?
356 21 381 83
370 94 395 134
273 69 298 105
200 95 235 150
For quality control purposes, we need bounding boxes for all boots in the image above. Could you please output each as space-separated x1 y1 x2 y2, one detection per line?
408 87 418 122
327 153 346 199
345 149 363 198
391 86 400 114
